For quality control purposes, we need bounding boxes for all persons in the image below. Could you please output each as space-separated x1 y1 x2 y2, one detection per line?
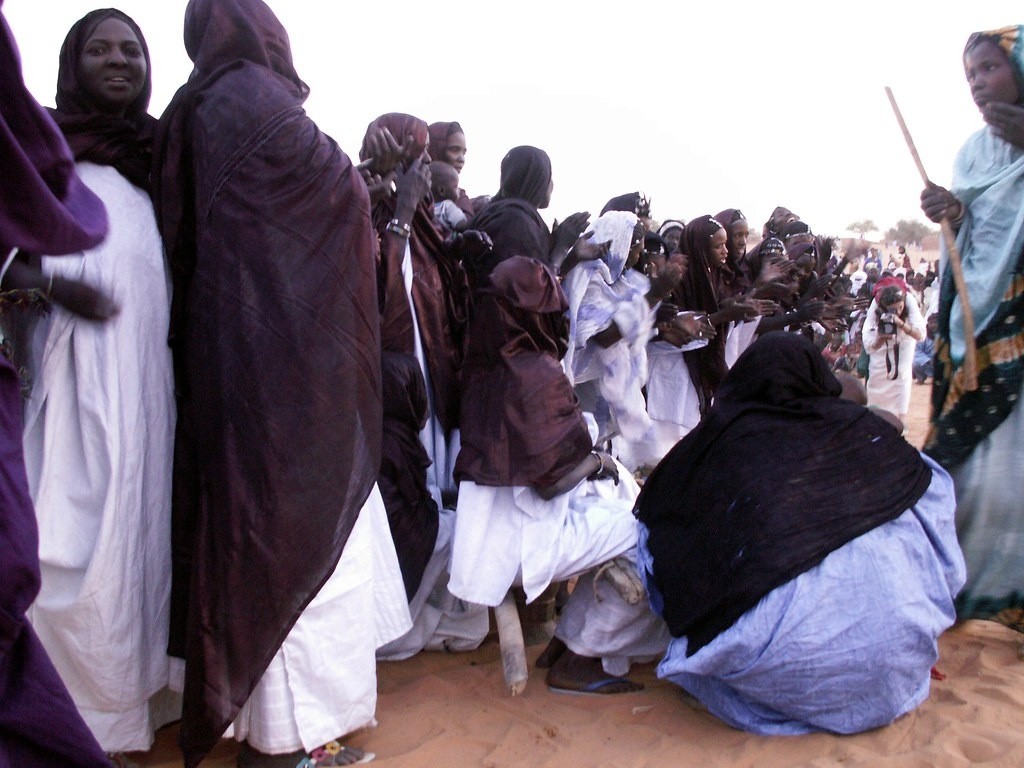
631 332 965 736
920 26 1024 635
358 113 493 660
0 0 414 768
428 121 939 695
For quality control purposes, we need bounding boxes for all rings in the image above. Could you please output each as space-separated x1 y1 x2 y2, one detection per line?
699 331 702 336
836 328 837 330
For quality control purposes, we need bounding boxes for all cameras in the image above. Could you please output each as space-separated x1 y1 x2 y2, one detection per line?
877 308 897 336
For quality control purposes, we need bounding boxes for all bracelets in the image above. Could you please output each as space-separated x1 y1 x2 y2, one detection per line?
387 219 411 237
549 265 561 275
900 321 904 328
708 314 713 327
951 203 966 228
591 450 603 475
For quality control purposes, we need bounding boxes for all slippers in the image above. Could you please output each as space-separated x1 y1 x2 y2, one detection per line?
239 738 374 767
547 673 648 697
